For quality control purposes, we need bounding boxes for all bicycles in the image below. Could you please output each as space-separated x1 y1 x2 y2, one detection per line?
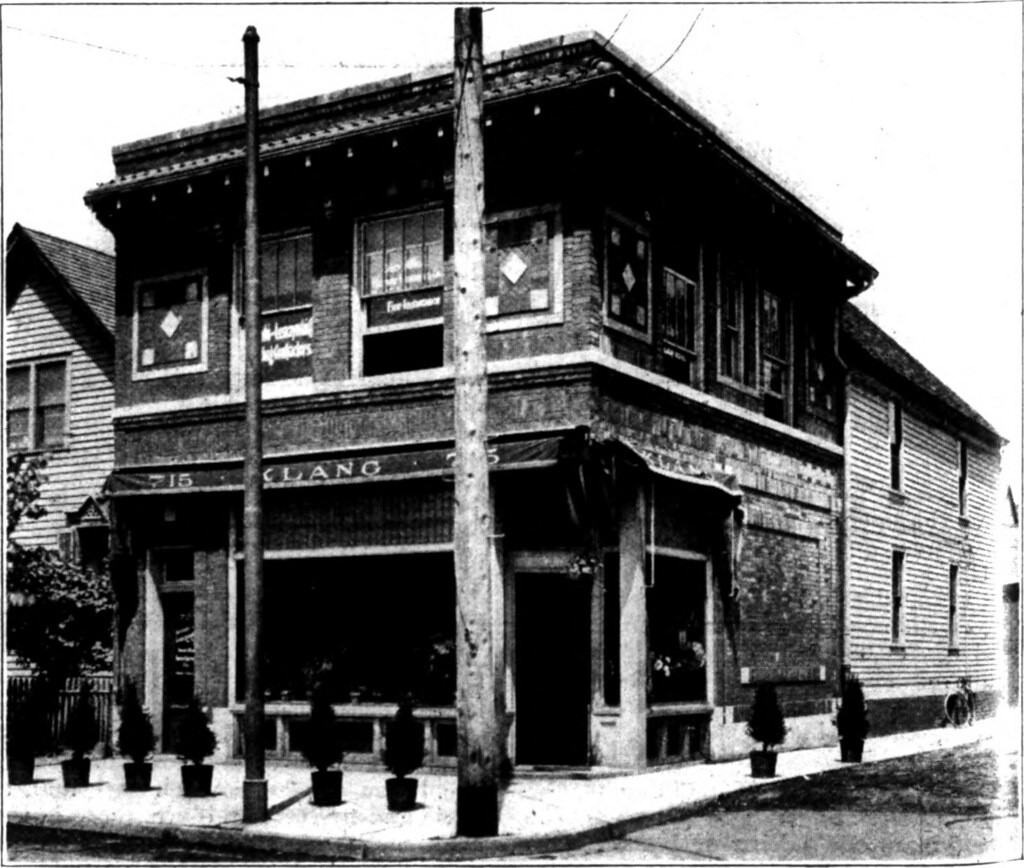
943 678 977 728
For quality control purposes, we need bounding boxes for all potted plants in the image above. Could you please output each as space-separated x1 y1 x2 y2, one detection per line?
830 664 870 763
300 684 345 807
7 678 51 786
175 696 215 798
380 694 426 813
745 681 789 779
60 680 100 788
116 673 159 792
